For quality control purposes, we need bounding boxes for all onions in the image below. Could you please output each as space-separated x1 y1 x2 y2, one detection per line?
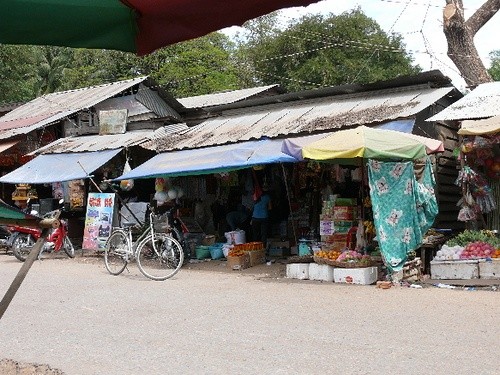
435 244 465 260
461 240 495 258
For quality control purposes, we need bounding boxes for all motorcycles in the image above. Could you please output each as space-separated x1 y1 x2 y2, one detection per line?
4 198 75 262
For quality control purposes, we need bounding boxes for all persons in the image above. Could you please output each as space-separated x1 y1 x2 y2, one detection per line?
249 180 272 241
212 211 252 243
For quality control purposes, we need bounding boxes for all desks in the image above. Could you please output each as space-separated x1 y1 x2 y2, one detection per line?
420 238 446 272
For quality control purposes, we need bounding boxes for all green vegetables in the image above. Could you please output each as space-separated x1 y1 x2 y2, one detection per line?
445 229 500 250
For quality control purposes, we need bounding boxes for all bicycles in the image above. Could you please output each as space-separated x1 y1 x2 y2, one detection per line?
104 203 185 281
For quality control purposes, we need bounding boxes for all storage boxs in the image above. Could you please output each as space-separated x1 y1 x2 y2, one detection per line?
333 267 378 285
286 263 308 280
320 206 354 250
430 259 478 280
479 258 500 279
309 263 333 282
244 251 266 267
269 241 289 257
226 255 250 269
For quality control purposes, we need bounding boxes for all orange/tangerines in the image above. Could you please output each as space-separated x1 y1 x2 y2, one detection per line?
317 251 342 259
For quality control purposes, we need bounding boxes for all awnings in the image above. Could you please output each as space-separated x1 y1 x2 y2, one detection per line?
105 119 417 182
0 148 124 183
0 140 20 153
